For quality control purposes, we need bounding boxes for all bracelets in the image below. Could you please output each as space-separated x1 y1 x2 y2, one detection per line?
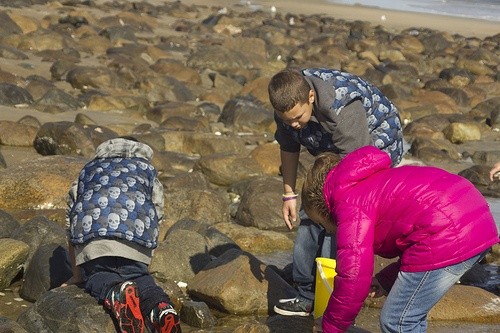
282 192 299 201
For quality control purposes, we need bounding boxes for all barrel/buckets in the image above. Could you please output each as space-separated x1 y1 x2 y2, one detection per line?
313 256 338 319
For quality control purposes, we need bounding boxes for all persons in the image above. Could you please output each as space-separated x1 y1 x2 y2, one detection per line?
300 145 500 333
269 68 403 316
65 136 182 333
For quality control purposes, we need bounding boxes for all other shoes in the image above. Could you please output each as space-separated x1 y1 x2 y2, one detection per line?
104 280 145 333
147 298 182 333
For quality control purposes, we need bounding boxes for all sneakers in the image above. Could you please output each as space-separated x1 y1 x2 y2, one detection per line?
273 298 313 316
